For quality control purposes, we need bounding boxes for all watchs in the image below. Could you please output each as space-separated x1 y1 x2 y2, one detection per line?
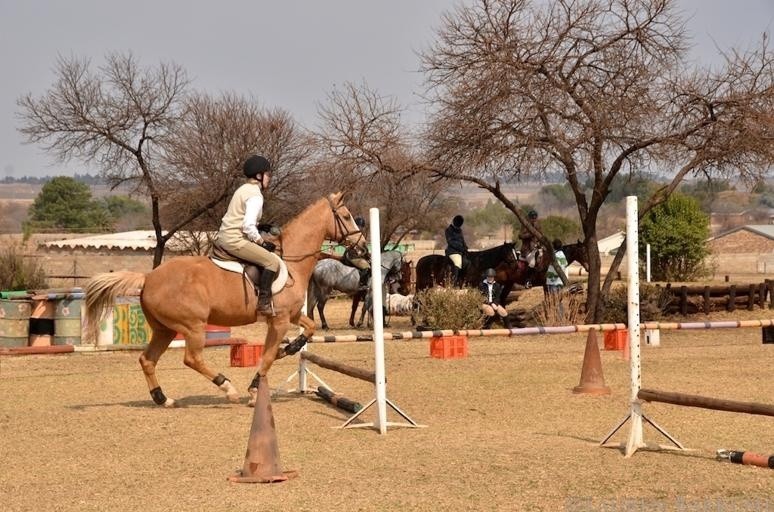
517 208 548 290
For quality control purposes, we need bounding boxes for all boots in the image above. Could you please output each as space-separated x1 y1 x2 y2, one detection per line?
502 314 513 329
256 270 276 306
483 315 493 329
360 268 369 299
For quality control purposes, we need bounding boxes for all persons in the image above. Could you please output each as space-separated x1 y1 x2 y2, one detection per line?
546 237 570 295
343 217 373 293
474 267 513 331
212 155 282 318
443 213 472 288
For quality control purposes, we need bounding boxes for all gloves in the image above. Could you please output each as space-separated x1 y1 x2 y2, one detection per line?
257 223 273 234
262 240 276 253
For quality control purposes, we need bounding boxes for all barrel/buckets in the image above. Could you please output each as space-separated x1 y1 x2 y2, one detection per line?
32 302 54 346
0 299 31 348
54 299 81 348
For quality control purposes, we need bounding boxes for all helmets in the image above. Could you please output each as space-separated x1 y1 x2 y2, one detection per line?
528 210 538 216
453 215 464 226
243 155 271 178
487 268 496 277
354 218 366 226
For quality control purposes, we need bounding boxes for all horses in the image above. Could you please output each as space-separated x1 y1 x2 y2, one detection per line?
306 249 412 332
412 240 521 329
77 187 370 411
493 238 590 311
